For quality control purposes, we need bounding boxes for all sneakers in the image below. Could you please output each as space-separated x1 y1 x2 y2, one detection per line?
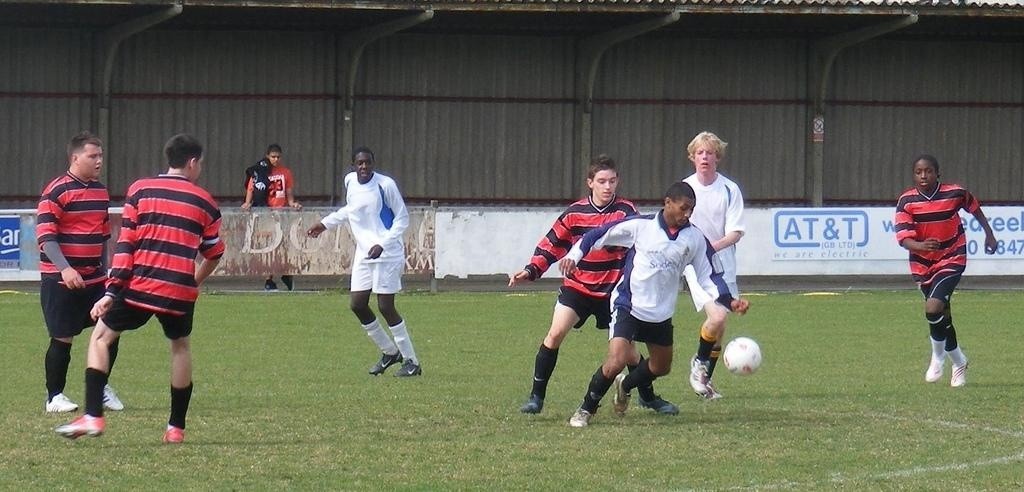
925 354 944 382
369 348 403 377
520 352 724 428
951 359 968 387
281 275 294 291
46 383 186 444
396 359 422 377
265 279 277 290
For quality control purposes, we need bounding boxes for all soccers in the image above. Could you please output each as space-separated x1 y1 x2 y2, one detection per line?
723 337 760 374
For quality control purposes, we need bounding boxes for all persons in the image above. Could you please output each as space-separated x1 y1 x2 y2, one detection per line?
54 133 225 443
558 181 750 427
508 153 680 416
37 131 125 413
895 155 997 388
307 146 422 377
241 143 302 290
681 131 745 400
